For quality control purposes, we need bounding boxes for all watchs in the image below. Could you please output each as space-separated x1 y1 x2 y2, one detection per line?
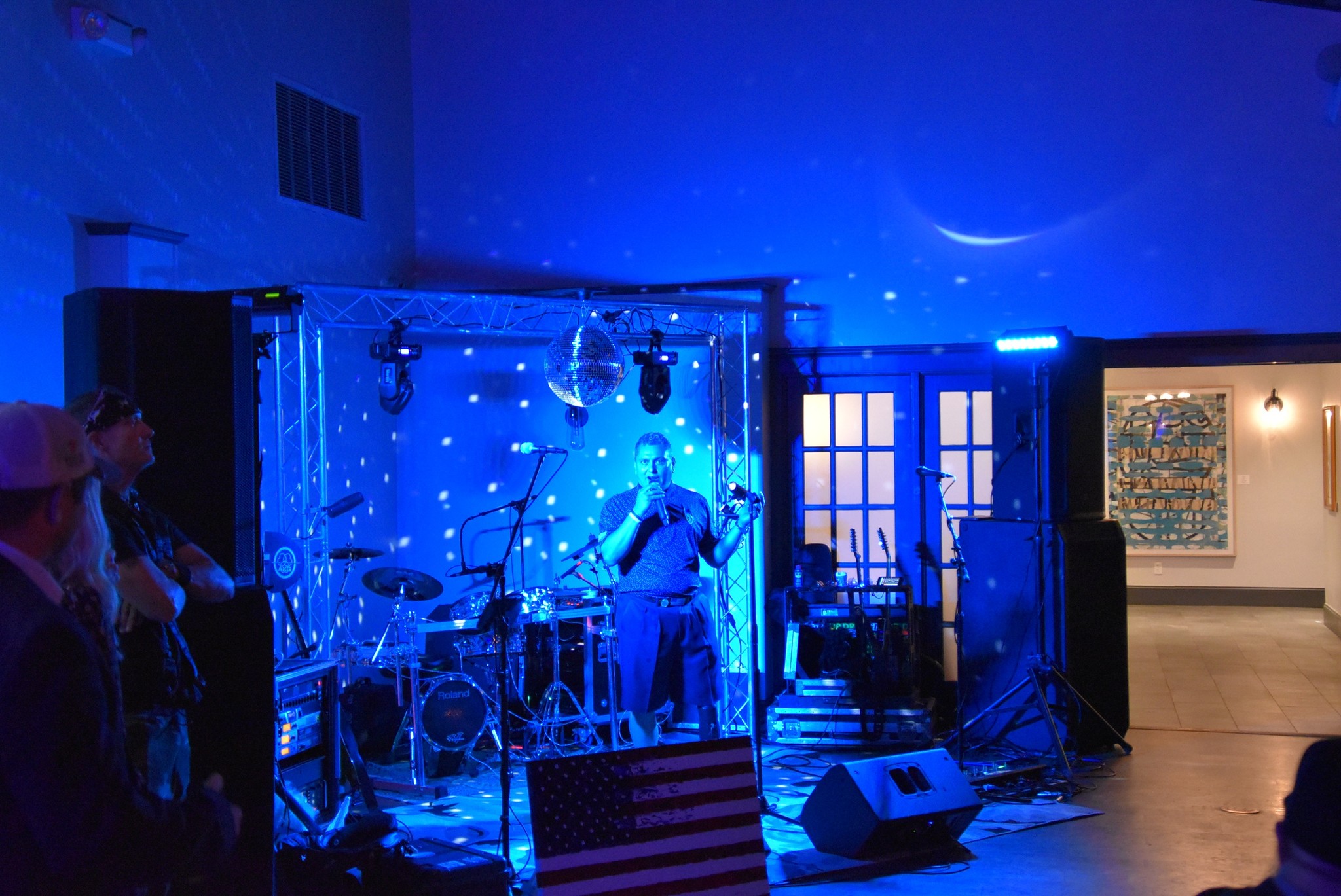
735 520 751 534
158 557 175 580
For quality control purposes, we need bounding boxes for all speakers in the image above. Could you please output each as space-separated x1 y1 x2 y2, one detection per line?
63 287 257 586
387 835 511 896
144 582 277 896
801 748 984 861
957 518 1130 756
991 359 1105 520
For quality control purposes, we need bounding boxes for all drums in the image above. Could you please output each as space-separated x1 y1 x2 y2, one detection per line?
417 673 490 751
447 591 502 637
505 586 558 627
355 639 416 666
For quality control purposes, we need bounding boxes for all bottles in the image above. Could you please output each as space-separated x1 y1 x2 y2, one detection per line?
794 565 803 587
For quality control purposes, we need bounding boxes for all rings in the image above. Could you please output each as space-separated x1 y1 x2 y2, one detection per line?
125 603 135 611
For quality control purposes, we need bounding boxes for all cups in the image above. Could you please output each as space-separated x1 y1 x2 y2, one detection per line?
835 572 847 587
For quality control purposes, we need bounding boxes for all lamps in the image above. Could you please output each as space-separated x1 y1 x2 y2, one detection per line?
1263 389 1284 414
544 324 624 450
633 330 678 415
935 325 1133 791
369 320 422 413
69 7 147 58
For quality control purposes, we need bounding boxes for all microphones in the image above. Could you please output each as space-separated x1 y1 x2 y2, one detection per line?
561 562 583 579
521 442 569 454
327 492 364 519
648 480 671 526
451 565 492 577
915 466 954 478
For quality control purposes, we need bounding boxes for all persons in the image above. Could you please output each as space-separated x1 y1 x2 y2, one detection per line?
0 383 241 896
599 432 765 747
1199 737 1341 896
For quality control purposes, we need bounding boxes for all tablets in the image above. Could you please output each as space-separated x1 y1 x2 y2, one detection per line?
877 576 903 586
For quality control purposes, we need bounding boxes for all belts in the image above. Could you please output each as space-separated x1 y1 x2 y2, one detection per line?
637 595 696 607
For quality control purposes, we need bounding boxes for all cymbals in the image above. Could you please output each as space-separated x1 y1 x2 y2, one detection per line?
361 567 444 602
549 587 589 599
313 546 386 559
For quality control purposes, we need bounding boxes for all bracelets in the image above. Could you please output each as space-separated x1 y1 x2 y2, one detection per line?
628 508 643 524
173 562 192 587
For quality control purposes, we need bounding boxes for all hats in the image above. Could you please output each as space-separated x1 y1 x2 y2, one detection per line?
66 389 136 432
0 401 106 488
1285 738 1341 867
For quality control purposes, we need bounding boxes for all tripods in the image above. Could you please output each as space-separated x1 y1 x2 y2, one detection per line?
937 361 1133 785
524 600 607 760
